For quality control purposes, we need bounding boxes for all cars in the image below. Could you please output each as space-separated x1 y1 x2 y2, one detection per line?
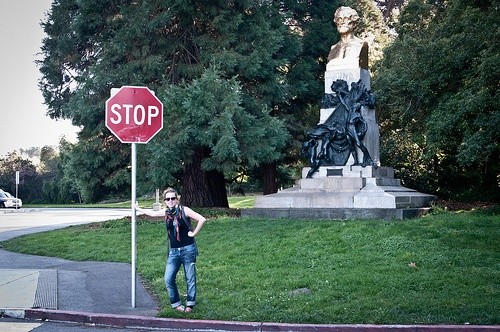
0 188 22 208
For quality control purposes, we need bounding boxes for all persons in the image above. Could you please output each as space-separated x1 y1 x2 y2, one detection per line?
304 138 321 179
134 187 206 313
336 91 374 166
328 6 369 66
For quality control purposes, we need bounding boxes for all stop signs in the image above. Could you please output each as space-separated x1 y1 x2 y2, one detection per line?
105 85 164 143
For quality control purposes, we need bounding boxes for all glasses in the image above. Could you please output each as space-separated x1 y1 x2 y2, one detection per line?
165 197 176 201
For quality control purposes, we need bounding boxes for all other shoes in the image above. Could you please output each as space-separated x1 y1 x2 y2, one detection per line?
175 305 185 311
185 307 193 313
